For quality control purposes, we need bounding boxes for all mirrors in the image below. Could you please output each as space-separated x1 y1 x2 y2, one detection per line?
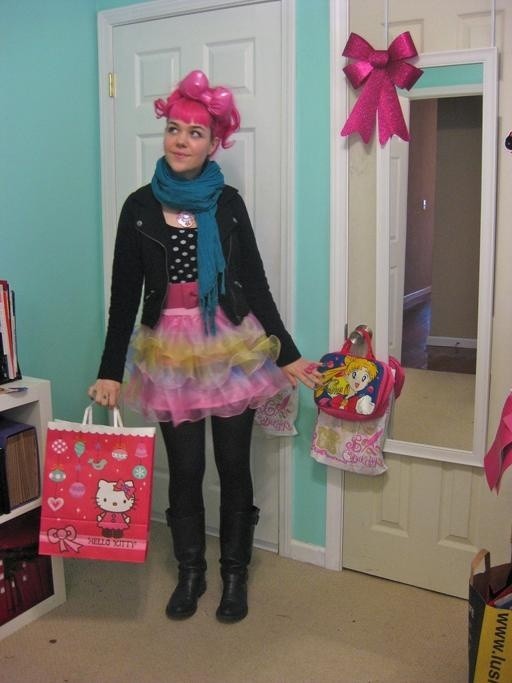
376 47 500 469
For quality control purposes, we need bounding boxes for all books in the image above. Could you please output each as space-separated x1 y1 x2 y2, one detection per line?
0 421 40 515
0 279 19 380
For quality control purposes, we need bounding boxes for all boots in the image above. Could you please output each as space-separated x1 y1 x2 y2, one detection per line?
165 506 206 620
216 505 260 622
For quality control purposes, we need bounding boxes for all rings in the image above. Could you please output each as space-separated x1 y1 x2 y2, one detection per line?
103 394 108 399
304 362 320 374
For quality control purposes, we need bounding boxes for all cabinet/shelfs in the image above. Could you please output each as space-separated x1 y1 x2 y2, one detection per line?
0 375 68 641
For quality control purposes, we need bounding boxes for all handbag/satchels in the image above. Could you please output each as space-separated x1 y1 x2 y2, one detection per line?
314 351 393 421
469 549 512 683
39 404 156 564
389 355 404 399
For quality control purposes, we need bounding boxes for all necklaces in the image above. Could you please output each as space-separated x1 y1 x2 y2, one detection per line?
176 209 196 228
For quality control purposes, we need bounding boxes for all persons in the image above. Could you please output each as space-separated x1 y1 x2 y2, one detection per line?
89 70 329 625
313 356 378 416
